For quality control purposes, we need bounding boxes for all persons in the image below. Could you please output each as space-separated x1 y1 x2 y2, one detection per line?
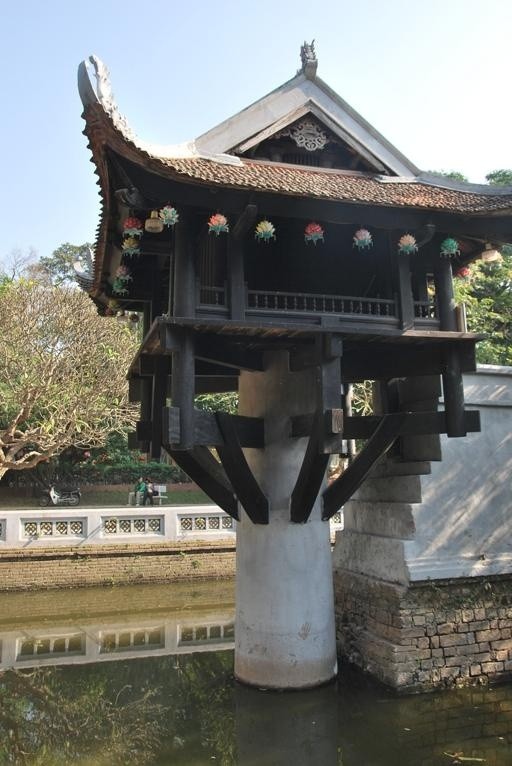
126 476 146 506
82 450 107 465
131 448 137 463
139 454 144 463
142 477 154 505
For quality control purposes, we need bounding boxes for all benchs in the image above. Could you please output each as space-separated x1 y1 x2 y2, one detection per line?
140 485 168 506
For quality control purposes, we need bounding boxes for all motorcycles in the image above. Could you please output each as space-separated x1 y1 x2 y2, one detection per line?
38 481 82 506
